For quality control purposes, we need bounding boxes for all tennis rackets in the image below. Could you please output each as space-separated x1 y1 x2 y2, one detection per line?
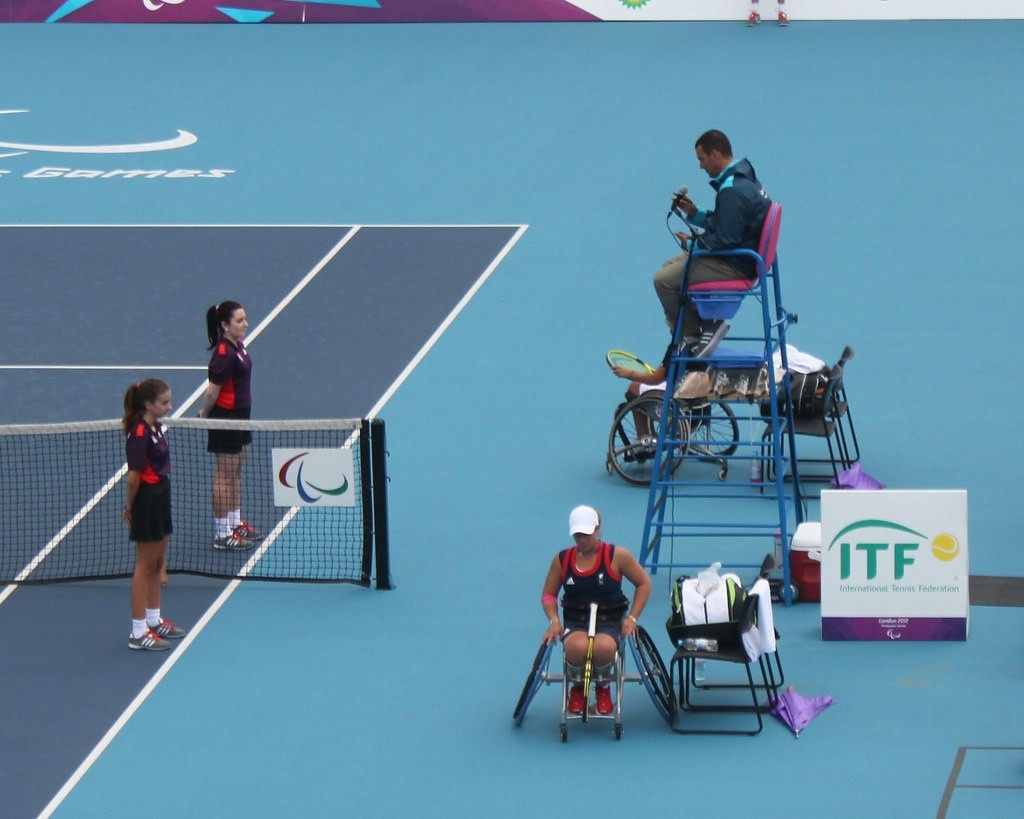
582 602 599 724
605 349 656 375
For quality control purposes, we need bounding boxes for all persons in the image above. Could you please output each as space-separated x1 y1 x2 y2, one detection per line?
542 505 651 716
654 130 771 359
121 378 186 650
197 301 263 550
746 0 790 26
613 319 712 457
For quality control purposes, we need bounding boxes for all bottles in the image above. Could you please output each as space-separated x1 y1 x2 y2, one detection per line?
751 450 761 482
774 530 783 570
678 638 718 651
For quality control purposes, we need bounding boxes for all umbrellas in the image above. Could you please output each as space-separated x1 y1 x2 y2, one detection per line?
764 685 833 733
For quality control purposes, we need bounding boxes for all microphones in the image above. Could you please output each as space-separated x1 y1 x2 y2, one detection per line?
667 186 688 219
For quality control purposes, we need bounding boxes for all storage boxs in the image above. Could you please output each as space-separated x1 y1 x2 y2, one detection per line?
666 615 740 649
789 520 822 603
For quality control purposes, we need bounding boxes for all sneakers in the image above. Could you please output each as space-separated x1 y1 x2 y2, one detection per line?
128 630 171 650
596 684 613 716
689 318 731 360
234 521 264 540
147 618 186 638
213 530 254 550
569 687 584 713
778 11 789 26
747 11 760 26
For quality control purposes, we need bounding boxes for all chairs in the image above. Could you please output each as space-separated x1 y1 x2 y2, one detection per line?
687 201 782 295
669 574 785 736
760 364 847 500
772 345 860 478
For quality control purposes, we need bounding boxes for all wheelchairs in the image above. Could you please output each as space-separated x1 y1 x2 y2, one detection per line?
511 604 680 743
606 390 740 488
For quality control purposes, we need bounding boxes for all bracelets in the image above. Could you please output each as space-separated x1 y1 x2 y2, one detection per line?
629 371 634 379
628 614 637 624
548 617 560 624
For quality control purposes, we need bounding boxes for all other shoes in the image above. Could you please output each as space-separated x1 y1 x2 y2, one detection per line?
626 439 655 464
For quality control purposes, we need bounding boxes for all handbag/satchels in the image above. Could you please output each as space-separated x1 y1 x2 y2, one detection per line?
759 366 833 425
671 574 747 626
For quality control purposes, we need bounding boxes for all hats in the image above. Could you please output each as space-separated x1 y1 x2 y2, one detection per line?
569 505 600 535
